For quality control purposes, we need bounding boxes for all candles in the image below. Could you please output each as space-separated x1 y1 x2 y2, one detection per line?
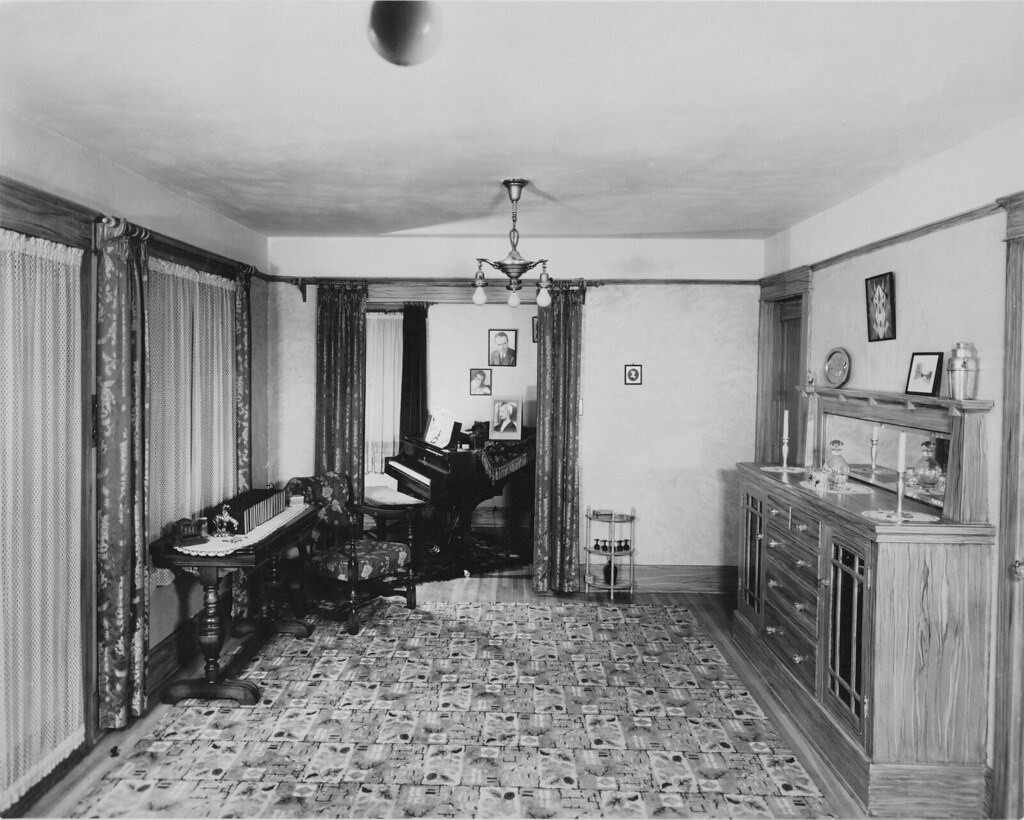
783 410 789 438
873 425 878 441
897 430 907 472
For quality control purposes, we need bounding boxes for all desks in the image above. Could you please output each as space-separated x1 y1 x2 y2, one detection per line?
148 495 317 705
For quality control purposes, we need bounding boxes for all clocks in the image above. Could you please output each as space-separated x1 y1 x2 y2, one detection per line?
822 348 849 386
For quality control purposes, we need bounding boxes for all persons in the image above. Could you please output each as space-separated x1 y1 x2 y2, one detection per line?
494 404 516 432
471 371 490 394
491 332 515 365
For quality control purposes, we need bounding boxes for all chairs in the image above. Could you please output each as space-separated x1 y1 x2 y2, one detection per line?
284 472 417 638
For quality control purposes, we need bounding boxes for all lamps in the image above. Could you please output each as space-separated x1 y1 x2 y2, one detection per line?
470 178 553 307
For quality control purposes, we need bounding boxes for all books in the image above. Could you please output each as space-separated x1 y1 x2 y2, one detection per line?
207 489 285 535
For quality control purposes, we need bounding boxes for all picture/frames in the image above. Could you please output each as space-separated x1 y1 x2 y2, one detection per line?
624 364 642 385
865 271 896 342
470 369 492 396
488 329 518 366
489 395 524 440
532 317 538 343
906 352 943 396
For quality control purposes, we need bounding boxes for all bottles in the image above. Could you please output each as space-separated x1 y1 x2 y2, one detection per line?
913 440 942 488
824 440 849 484
947 342 977 400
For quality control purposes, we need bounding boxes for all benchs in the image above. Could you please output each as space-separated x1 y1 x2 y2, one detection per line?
364 486 425 541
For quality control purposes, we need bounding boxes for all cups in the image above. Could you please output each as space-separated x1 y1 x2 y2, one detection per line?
808 466 847 491
903 467 946 495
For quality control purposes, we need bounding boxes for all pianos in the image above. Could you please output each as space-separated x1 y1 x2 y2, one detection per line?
384 415 535 566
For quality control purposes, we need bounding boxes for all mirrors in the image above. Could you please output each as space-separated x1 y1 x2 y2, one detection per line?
823 413 952 509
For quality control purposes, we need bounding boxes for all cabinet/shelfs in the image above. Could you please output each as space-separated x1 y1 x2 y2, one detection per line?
727 463 996 820
584 506 636 600
